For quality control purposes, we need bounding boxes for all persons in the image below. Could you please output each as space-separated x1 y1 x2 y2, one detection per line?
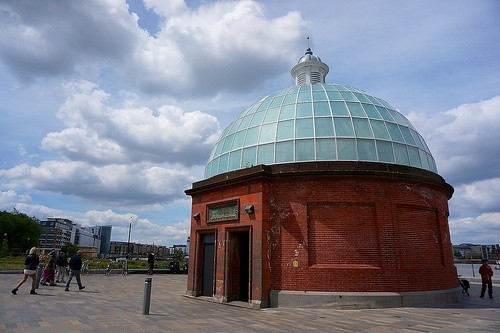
168 258 189 274
11 246 86 295
479 259 493 298
147 251 155 275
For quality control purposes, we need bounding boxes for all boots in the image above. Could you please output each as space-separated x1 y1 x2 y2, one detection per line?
30 289 37 295
11 288 18 296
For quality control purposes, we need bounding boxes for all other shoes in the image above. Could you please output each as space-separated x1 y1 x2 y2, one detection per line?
65 287 70 291
61 279 66 283
79 286 86 290
50 283 57 285
42 282 48 286
480 295 485 297
490 295 494 298
55 280 60 282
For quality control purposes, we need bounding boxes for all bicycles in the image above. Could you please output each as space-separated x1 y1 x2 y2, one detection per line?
82 261 92 276
458 274 470 297
105 264 113 277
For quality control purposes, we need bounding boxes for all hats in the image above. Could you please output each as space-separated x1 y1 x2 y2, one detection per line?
482 259 488 263
